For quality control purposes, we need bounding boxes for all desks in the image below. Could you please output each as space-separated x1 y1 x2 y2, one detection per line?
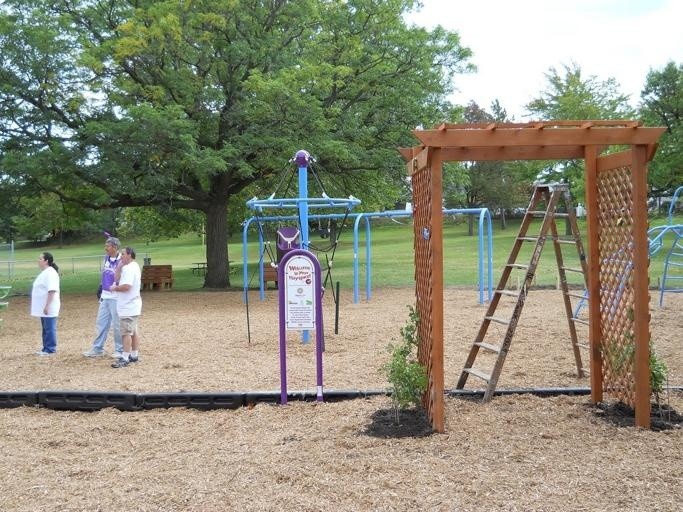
192 262 206 276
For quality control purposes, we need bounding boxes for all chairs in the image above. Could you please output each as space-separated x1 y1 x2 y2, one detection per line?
260 263 278 289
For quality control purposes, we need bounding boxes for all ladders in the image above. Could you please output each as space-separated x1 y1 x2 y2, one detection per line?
456 183 618 399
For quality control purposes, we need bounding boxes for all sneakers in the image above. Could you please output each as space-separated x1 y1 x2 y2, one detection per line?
35 350 138 368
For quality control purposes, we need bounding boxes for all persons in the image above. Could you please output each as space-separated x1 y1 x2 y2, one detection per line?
31 253 61 355
83 237 142 367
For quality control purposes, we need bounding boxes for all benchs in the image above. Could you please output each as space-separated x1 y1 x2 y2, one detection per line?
190 267 207 275
140 265 172 290
